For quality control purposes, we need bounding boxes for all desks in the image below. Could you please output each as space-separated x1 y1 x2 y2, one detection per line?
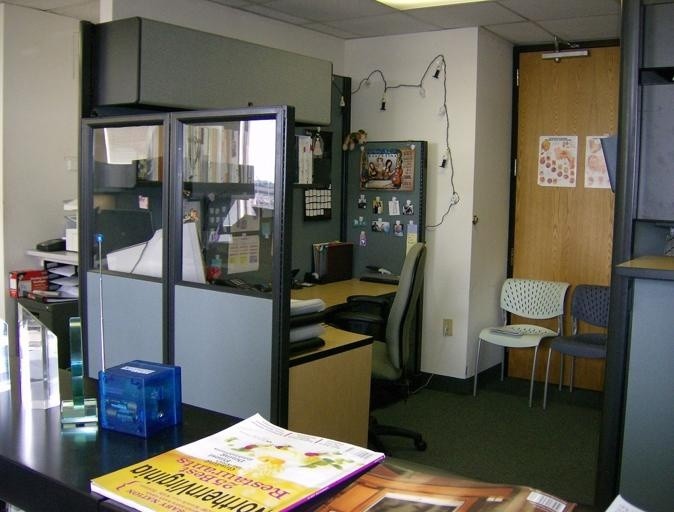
0 355 245 511
288 277 398 448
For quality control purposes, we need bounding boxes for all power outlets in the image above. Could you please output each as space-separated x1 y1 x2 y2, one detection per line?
443 319 453 336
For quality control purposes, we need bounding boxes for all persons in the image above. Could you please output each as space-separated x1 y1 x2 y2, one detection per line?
369 157 395 179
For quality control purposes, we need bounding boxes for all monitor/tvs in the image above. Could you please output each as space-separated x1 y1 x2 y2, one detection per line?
107 222 207 284
100 209 154 258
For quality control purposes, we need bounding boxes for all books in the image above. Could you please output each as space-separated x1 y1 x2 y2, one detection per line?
308 456 578 512
90 411 387 512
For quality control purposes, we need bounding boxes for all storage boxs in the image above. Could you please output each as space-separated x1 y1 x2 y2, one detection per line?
98 360 181 438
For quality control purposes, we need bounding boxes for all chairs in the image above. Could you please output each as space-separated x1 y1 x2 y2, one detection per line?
473 278 571 408
343 242 427 452
543 284 611 409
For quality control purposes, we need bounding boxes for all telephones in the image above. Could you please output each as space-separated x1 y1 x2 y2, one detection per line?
214 276 249 288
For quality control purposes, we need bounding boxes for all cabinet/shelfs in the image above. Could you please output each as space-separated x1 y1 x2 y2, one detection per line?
504 39 621 394
